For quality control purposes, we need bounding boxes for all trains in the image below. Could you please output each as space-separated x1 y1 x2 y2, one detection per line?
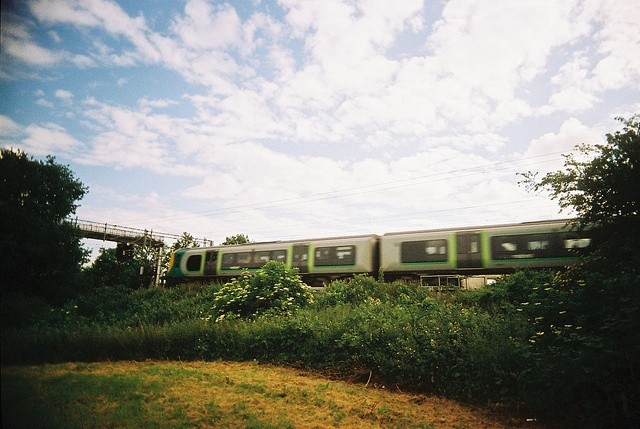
159 216 603 282
